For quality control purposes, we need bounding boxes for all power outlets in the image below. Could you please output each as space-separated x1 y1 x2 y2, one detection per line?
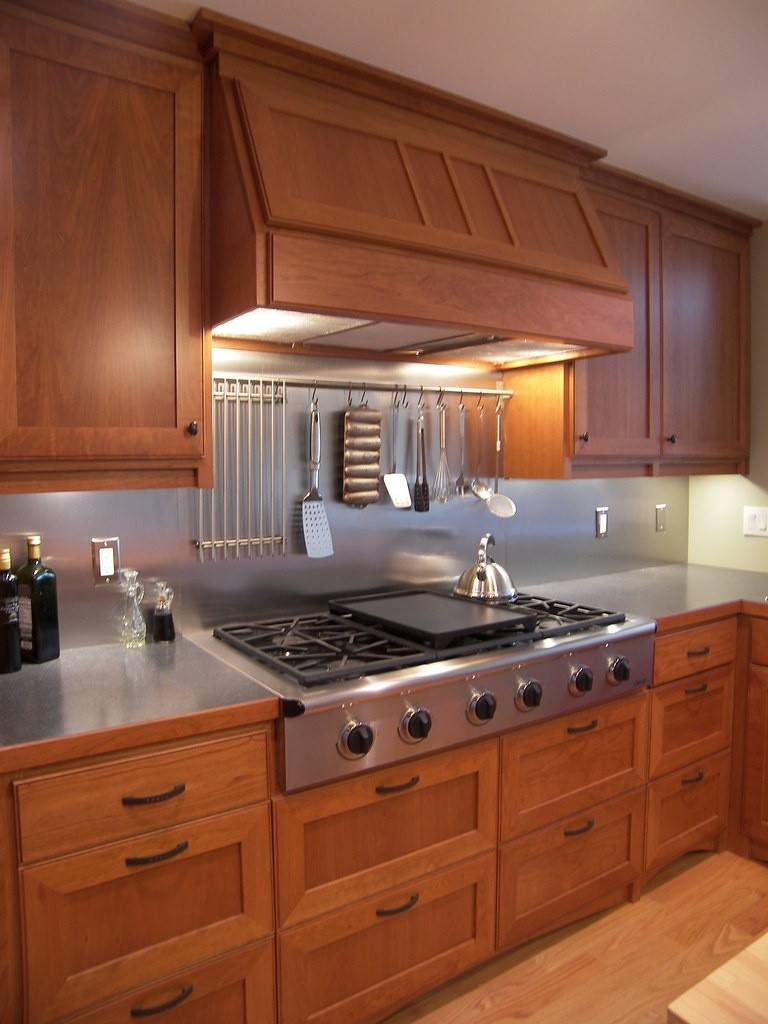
596 507 609 539
91 537 121 588
656 504 667 532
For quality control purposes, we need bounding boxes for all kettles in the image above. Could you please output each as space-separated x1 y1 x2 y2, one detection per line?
453 533 518 605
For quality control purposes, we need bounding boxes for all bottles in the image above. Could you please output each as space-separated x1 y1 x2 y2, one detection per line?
15 536 60 664
0 548 22 674
152 582 174 643
122 571 146 649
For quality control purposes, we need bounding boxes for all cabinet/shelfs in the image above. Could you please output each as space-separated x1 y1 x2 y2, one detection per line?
465 161 763 480
0 599 768 1024
0 0 217 495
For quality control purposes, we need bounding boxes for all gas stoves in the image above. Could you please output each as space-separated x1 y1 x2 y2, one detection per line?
182 585 658 794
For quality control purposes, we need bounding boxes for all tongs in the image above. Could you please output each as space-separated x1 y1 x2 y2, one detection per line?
415 416 429 512
